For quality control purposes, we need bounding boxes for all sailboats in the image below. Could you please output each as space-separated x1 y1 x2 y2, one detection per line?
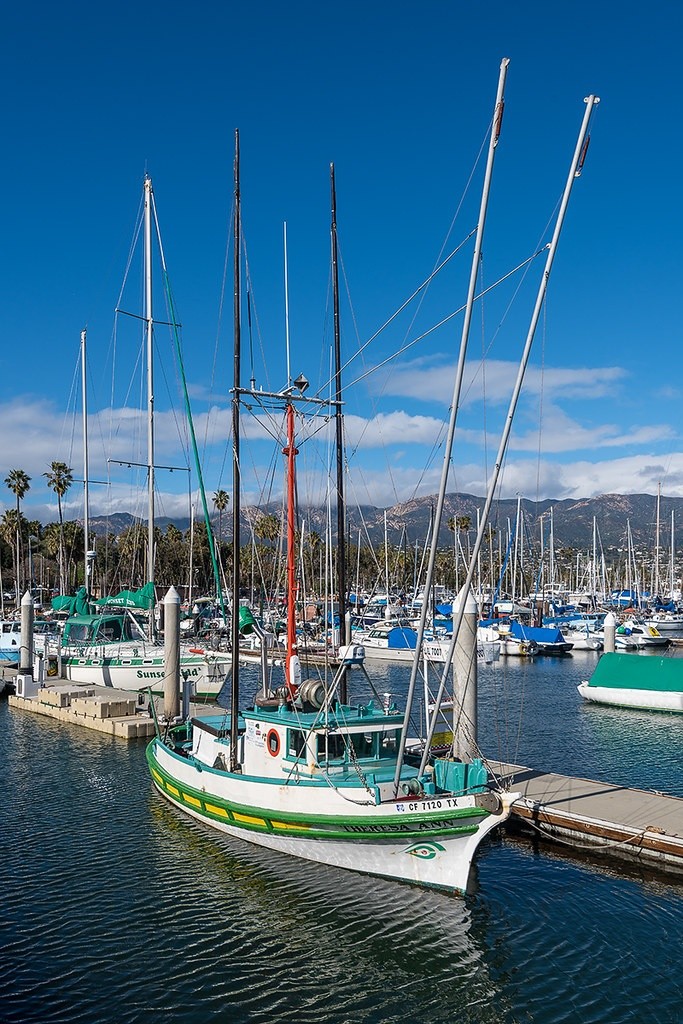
0 172 683 714
142 57 604 898
